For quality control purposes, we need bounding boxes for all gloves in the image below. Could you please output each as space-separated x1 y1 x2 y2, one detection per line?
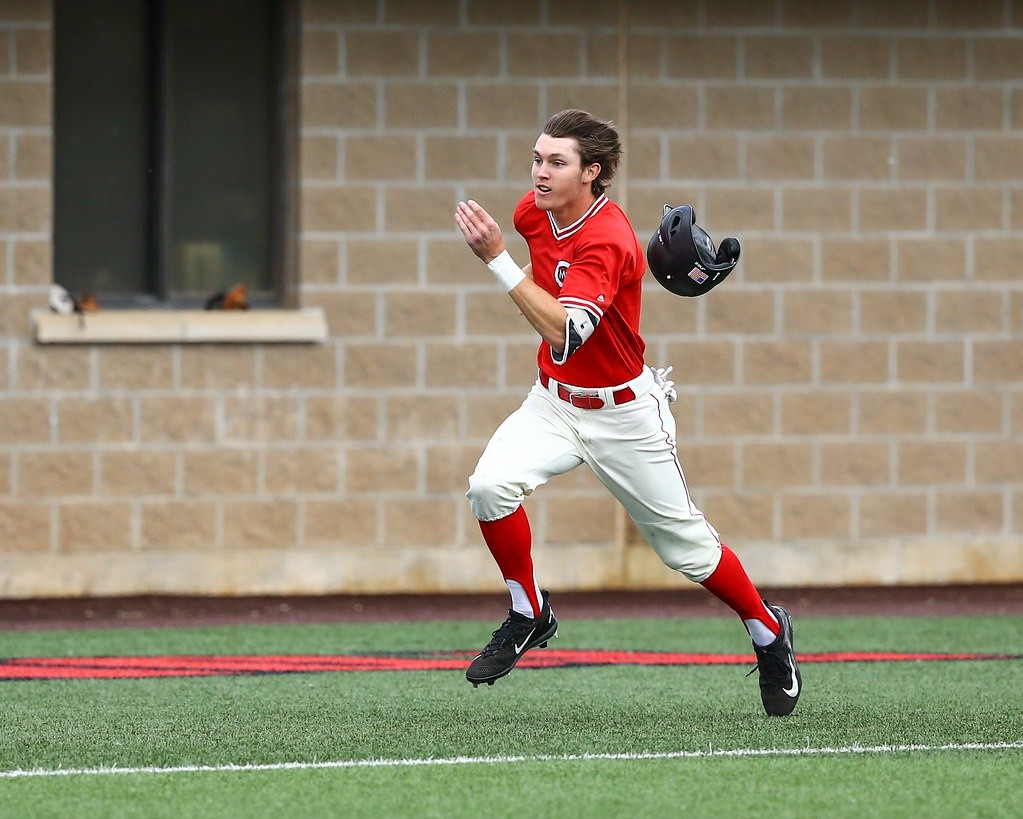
651 366 677 403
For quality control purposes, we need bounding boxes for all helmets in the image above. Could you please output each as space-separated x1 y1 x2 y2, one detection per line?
647 203 742 297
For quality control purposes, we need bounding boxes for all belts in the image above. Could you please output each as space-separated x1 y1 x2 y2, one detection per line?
539 370 635 409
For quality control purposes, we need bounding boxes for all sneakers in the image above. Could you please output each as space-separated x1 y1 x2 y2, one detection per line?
465 589 558 688
745 598 802 716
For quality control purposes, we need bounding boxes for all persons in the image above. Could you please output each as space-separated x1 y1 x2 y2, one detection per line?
453 108 802 716
